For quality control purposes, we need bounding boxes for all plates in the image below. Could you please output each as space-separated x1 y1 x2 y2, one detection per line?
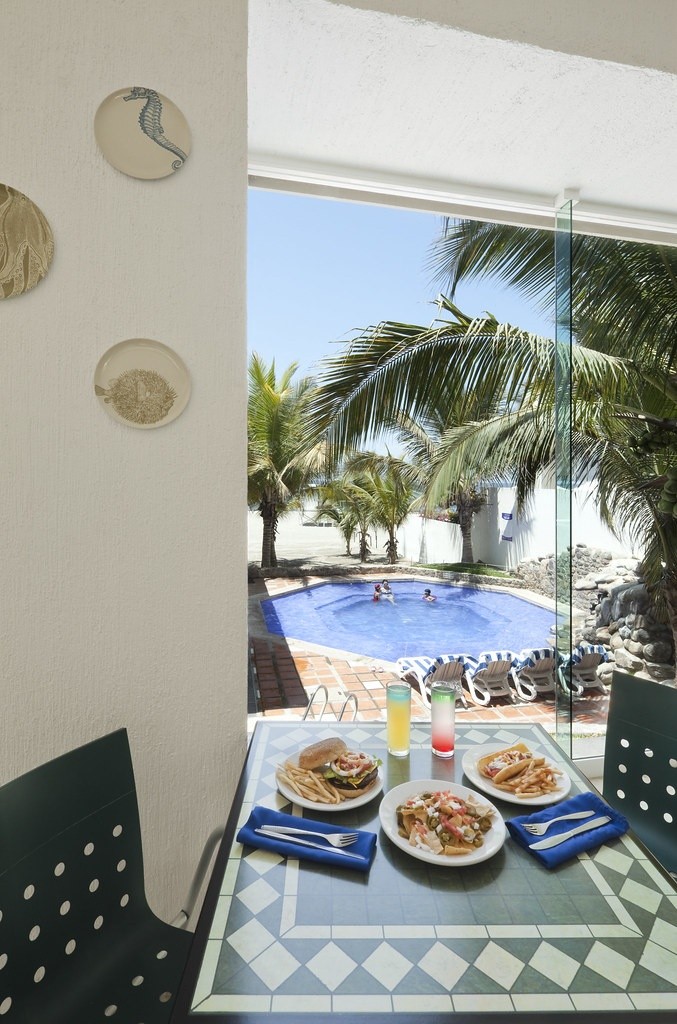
380 780 506 866
275 751 383 811
462 743 571 805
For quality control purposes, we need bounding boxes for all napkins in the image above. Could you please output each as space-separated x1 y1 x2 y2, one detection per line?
235 806 376 872
504 793 630 869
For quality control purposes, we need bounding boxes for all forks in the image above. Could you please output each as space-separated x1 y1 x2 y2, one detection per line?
261 824 359 848
520 810 595 836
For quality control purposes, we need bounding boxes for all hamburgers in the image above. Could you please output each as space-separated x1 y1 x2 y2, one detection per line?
299 737 384 797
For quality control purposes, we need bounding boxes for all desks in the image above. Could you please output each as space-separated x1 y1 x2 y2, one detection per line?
171 719 677 1024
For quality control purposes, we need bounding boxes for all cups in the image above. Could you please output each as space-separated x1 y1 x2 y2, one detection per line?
431 687 456 758
386 682 411 757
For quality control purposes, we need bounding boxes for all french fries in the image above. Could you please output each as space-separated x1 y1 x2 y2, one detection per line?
276 760 346 803
492 762 563 797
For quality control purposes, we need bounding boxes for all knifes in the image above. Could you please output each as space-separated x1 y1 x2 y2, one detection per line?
529 816 612 850
253 828 366 860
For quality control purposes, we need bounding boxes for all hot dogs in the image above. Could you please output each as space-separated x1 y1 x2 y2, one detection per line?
478 742 545 783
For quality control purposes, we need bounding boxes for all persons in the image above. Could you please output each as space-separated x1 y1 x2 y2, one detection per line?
380 580 391 592
374 584 381 601
424 589 434 599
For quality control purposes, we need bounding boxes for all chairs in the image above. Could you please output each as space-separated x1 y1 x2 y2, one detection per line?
439 654 516 706
479 647 563 700
520 645 608 697
599 671 676 883
1 726 193 1024
395 654 469 711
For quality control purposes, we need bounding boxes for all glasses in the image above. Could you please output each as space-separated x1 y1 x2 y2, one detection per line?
383 583 389 585
377 587 381 590
